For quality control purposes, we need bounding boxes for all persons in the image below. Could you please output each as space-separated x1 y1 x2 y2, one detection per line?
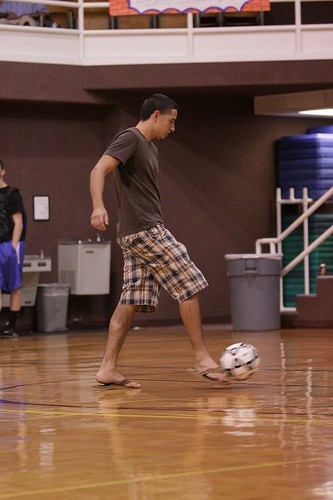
90 95 227 389
0 160 27 337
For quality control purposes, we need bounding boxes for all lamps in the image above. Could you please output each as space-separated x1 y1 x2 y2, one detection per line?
254 87 333 119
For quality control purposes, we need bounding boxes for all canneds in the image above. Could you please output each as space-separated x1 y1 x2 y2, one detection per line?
320 264 326 275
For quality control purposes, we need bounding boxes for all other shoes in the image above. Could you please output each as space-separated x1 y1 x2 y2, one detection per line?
1 325 15 338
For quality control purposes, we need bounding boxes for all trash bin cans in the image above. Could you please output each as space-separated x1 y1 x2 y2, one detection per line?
36 282 73 333
223 252 284 332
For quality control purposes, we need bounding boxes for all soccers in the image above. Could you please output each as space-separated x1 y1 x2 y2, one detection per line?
220 343 262 383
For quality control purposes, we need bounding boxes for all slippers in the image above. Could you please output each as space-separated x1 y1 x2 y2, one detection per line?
193 364 232 385
92 375 138 389
